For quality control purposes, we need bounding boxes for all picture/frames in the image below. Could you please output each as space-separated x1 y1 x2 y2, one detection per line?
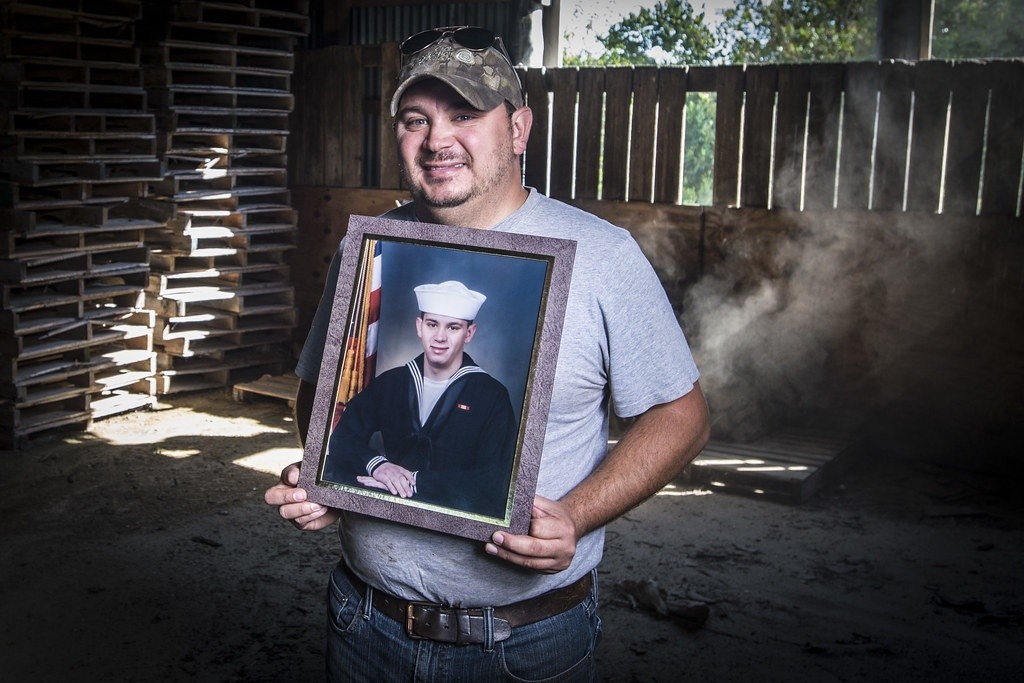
296 214 578 544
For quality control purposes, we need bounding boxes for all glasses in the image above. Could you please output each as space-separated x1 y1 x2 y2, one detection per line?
398 26 524 99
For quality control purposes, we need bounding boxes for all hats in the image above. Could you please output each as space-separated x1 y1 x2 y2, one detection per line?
414 280 487 321
389 27 523 111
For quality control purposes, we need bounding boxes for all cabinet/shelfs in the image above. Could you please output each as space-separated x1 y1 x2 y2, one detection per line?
0 0 310 445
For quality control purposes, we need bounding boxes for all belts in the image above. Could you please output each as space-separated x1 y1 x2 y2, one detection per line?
343 559 593 645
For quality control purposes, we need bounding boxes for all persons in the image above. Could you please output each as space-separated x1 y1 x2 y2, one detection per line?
263 23 714 682
328 280 518 521
